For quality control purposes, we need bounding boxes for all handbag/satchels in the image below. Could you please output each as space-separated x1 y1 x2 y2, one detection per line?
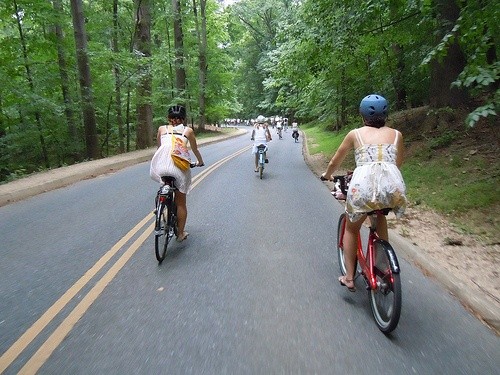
170 135 192 172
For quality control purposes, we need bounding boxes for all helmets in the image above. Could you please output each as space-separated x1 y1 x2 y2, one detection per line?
257 114 266 124
359 94 388 124
167 105 186 120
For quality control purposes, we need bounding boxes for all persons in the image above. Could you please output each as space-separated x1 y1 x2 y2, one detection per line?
150 104 204 242
226 115 299 172
322 95 407 292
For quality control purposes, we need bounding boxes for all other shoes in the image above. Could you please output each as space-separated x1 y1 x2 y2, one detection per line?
264 158 268 163
255 168 259 172
176 231 189 243
152 208 157 214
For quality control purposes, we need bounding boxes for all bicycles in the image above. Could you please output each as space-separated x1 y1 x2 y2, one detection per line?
251 137 271 180
318 170 400 329
293 131 302 142
277 127 282 141
153 163 204 265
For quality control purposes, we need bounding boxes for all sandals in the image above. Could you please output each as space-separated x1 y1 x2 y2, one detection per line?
337 276 357 292
